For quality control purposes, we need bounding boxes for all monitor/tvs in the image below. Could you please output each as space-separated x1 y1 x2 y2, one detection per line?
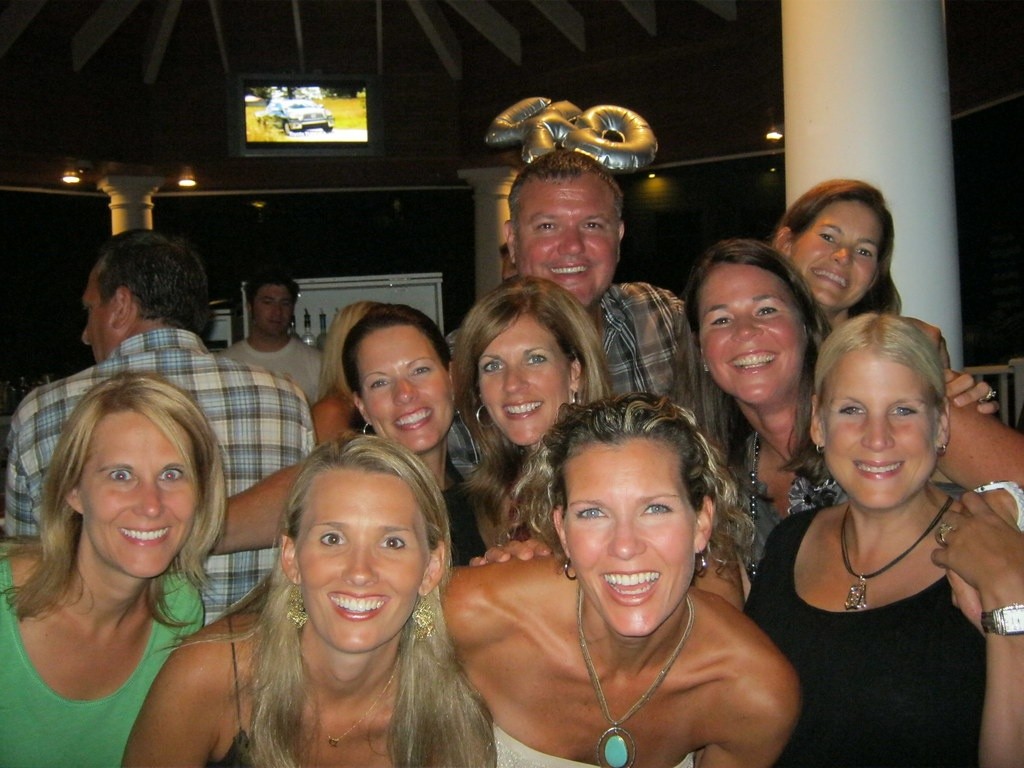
227 73 384 160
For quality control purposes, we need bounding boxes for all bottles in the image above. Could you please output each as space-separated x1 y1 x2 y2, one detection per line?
316 313 328 350
289 316 300 341
302 313 316 347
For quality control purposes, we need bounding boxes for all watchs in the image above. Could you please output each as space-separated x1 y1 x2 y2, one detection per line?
981 603 1024 636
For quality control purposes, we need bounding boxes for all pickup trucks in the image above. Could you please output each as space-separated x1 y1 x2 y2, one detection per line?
254 97 335 136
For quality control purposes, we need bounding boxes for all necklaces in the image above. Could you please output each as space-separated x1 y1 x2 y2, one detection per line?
748 432 761 578
303 656 399 748
840 495 953 611
577 585 695 768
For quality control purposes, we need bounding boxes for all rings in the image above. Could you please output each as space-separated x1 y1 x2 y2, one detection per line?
977 386 997 403
485 544 502 564
938 523 953 544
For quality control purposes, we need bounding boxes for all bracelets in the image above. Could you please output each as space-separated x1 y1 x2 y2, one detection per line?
972 480 1024 532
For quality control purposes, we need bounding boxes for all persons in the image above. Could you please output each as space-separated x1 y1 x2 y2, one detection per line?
4 228 318 627
207 303 487 567
0 370 226 768
310 301 386 445
452 274 614 567
668 238 1024 613
766 178 1000 414
217 270 322 408
439 390 804 768
686 314 1024 768
119 427 498 768
503 150 740 467
